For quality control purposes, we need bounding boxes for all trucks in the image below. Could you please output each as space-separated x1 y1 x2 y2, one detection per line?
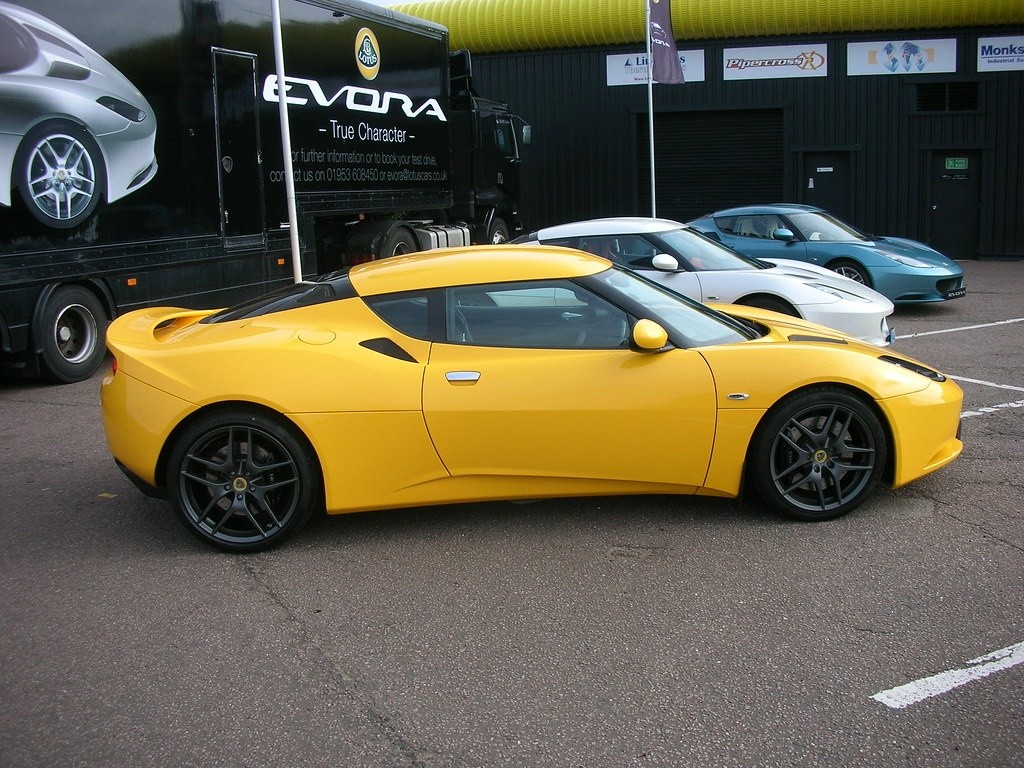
0 0 533 389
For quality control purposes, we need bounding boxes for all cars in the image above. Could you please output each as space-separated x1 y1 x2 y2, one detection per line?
1 3 160 234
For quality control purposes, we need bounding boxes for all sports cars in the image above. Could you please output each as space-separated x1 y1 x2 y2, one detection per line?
621 203 967 306
410 216 900 348
96 245 965 554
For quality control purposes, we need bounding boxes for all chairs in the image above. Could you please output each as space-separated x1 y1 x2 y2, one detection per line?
588 239 618 262
733 217 763 238
766 217 778 239
